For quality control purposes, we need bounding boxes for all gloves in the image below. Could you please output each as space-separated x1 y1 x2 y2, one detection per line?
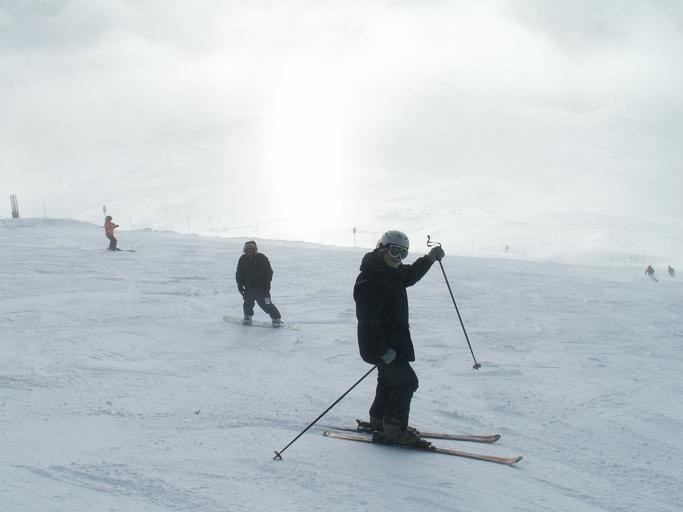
239 288 244 295
380 348 397 364
428 246 445 261
264 283 270 290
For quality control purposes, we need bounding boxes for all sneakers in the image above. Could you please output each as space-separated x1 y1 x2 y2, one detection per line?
272 319 281 327
370 415 431 446
243 315 255 324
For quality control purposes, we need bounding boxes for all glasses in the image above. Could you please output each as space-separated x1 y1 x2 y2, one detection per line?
388 245 408 260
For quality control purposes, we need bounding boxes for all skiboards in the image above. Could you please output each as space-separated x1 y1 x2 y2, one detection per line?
324 423 522 464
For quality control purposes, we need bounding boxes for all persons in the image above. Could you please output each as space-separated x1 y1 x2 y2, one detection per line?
105 216 117 250
668 266 674 276
235 240 281 326
353 230 445 448
645 265 655 276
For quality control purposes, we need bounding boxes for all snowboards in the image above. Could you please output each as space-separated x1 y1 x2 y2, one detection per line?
223 314 300 330
106 248 136 253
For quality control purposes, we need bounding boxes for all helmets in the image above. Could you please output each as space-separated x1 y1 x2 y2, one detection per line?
245 241 257 249
376 230 409 251
